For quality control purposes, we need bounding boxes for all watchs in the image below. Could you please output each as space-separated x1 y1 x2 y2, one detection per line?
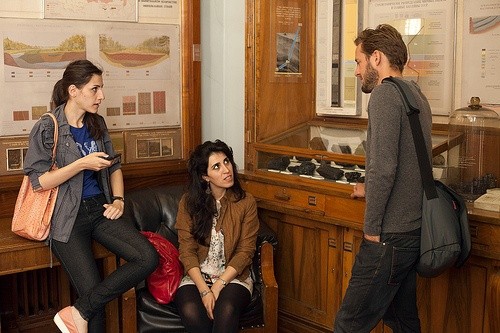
219 277 227 286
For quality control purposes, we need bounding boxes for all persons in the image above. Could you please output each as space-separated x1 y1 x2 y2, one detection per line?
23 59 160 333
331 22 433 333
172 138 260 333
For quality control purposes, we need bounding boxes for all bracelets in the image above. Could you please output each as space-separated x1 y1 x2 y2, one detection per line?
113 195 125 202
199 288 212 297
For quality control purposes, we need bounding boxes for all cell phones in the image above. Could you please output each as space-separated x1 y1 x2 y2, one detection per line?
105 153 122 161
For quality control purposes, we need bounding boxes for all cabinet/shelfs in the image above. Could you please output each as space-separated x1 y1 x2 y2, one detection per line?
236 120 500 333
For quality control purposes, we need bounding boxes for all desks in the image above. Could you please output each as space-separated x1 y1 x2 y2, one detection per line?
0 216 119 333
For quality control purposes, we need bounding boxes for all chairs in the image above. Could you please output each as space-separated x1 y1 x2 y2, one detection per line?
121 175 278 333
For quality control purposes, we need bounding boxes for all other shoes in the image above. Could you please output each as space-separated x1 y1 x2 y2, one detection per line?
53 306 78 333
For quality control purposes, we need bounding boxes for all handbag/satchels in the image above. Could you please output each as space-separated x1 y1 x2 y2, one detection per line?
419 179 472 277
11 112 58 241
140 230 183 305
